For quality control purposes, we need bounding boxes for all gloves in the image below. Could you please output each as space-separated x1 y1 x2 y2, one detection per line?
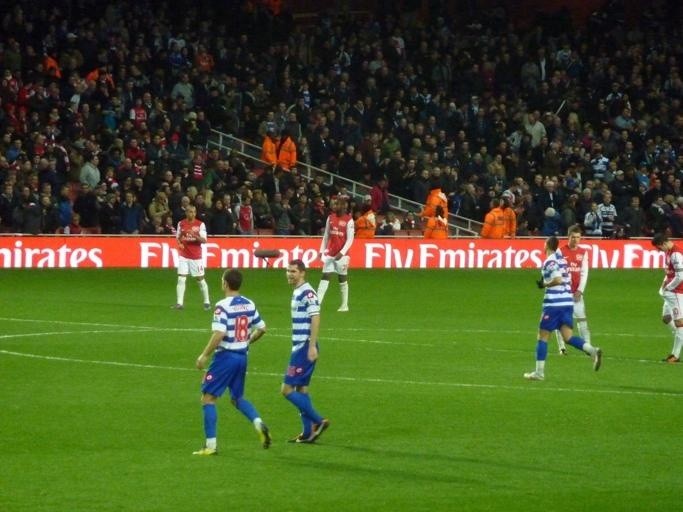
536 277 545 288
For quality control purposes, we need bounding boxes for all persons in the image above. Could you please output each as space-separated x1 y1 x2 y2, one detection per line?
188 267 275 456
553 224 593 359
649 231 683 362
167 203 212 312
2 1 683 238
522 234 603 382
311 198 357 313
278 259 331 443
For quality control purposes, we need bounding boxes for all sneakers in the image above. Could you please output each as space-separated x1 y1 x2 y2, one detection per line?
257 422 271 449
523 371 545 381
560 348 568 355
203 303 211 312
169 303 185 310
309 419 330 443
337 307 349 311
288 433 308 444
193 447 217 456
661 352 680 362
591 347 602 373
